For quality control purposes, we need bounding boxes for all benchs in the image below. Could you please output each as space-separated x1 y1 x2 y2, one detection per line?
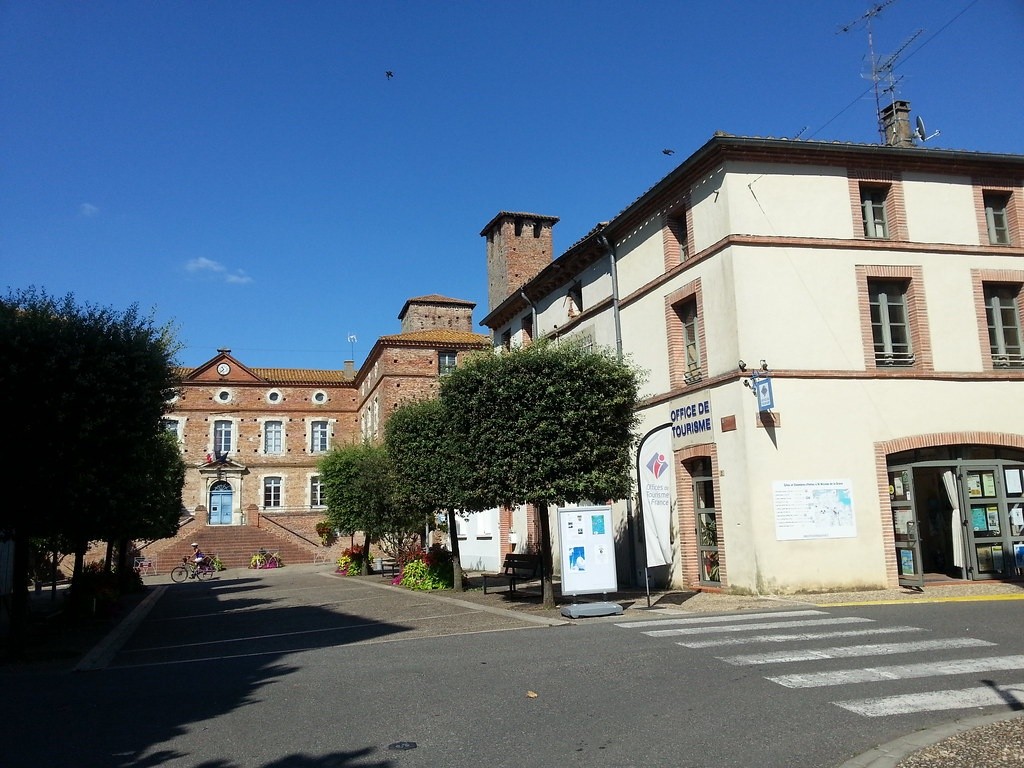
380 558 400 578
481 554 539 604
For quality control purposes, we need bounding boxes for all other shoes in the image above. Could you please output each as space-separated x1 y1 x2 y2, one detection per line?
189 576 195 579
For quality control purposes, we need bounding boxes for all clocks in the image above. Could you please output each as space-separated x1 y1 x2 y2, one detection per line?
217 363 231 376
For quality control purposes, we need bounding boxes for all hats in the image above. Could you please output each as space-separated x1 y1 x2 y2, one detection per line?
191 543 198 546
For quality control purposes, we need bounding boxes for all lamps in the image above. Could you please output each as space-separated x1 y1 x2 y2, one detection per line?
760 360 768 368
738 360 746 369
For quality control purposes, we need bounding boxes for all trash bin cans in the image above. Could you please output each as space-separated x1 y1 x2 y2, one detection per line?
372 557 383 574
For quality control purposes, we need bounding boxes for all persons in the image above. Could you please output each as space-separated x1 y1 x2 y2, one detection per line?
183 543 204 579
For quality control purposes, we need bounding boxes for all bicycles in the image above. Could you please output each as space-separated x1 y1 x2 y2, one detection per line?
171 556 214 583
